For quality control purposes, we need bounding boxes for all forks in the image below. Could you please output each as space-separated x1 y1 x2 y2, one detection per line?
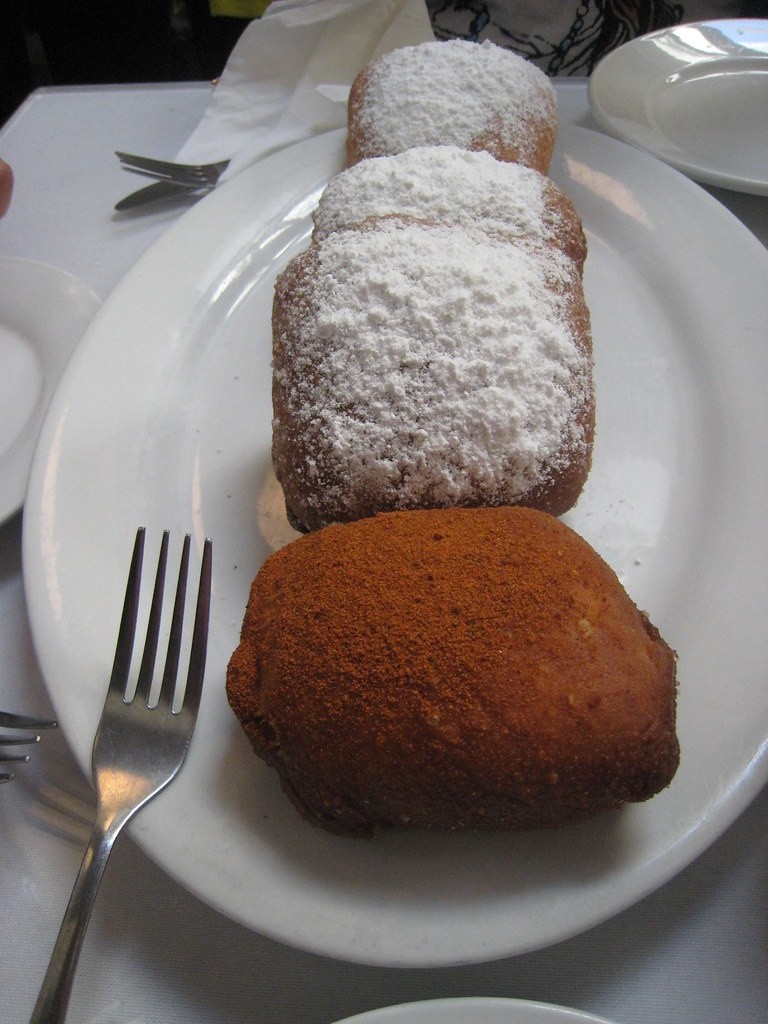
116 149 228 187
1 712 58 782
26 526 214 1022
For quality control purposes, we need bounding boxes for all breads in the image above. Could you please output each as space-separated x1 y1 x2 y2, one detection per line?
222 36 686 835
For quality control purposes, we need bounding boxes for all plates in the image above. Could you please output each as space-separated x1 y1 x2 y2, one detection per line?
2 255 102 529
26 112 768 967
589 16 766 195
333 996 612 1024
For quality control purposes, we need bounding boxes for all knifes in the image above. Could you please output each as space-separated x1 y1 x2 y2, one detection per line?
115 182 207 212
